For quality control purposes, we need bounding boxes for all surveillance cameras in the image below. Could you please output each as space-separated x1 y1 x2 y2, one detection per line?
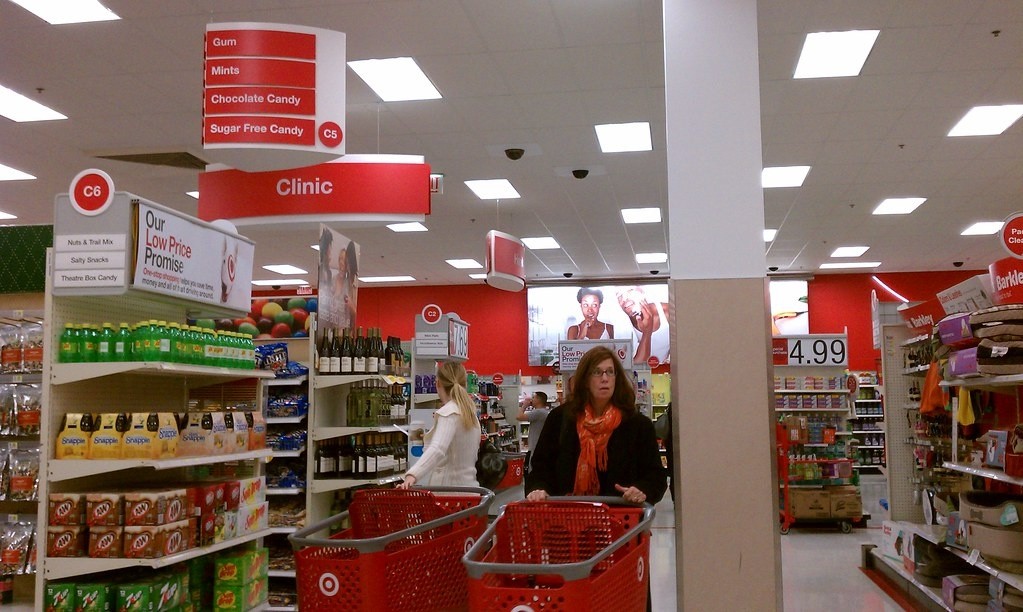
572 170 589 179
563 273 573 278
769 267 778 272
505 148 525 161
650 271 659 275
952 261 963 267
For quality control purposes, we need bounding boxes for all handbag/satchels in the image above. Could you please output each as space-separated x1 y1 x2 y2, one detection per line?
655 412 670 440
475 439 508 492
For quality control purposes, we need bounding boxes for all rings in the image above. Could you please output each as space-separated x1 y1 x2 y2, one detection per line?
634 496 638 499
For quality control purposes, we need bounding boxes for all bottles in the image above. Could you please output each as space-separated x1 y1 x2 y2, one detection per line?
58 319 256 369
314 432 407 480
775 413 847 481
346 379 410 427
318 326 405 375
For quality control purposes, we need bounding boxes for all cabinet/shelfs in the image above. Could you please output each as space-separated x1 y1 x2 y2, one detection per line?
633 372 656 423
774 360 861 520
0 191 518 612
924 368 1023 612
521 380 559 452
901 333 960 577
854 378 883 473
652 403 671 468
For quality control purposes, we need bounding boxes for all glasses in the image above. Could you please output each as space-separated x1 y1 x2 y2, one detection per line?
590 368 618 377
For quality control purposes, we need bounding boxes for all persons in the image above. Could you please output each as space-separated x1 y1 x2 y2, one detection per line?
516 391 548 451
318 228 358 326
566 288 614 341
395 362 482 497
523 347 667 612
618 288 671 361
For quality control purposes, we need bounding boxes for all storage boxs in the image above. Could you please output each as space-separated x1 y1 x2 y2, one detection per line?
793 491 830 518
226 508 238 538
775 374 847 410
788 488 794 516
831 492 865 517
214 481 226 513
217 544 272 586
46 526 84 556
194 514 216 543
46 582 76 612
826 484 857 493
49 491 84 527
86 490 125 527
239 473 267 506
117 571 193 612
122 486 189 527
125 517 195 556
238 501 272 538
215 573 270 611
90 524 123 556
75 583 114 612
986 428 1009 467
216 510 228 542
197 484 216 512
227 480 241 510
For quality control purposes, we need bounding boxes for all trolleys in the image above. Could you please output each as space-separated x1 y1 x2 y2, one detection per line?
460 495 657 612
289 485 497 612
